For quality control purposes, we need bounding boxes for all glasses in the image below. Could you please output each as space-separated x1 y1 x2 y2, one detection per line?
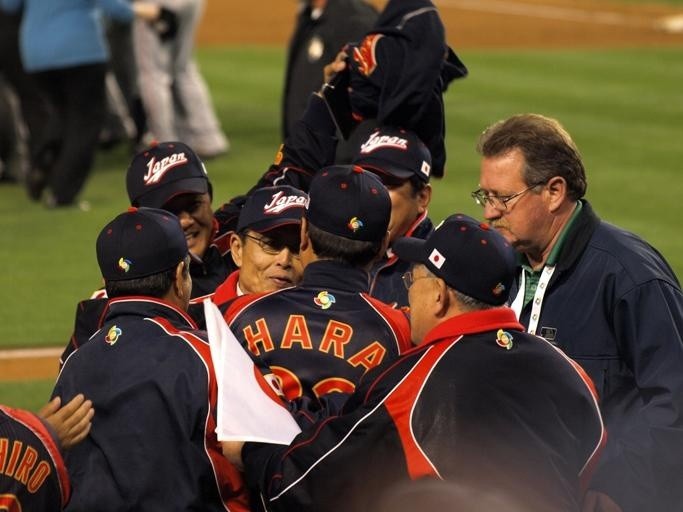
471 177 548 210
241 230 299 254
400 269 438 289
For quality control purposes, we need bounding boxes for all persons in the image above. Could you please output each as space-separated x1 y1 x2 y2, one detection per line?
283 1 465 184
0 0 232 207
1 114 682 510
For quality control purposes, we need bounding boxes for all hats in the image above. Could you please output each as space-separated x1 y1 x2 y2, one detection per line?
304 163 391 244
236 185 309 234
95 207 204 281
124 141 214 210
350 128 432 186
389 213 516 308
